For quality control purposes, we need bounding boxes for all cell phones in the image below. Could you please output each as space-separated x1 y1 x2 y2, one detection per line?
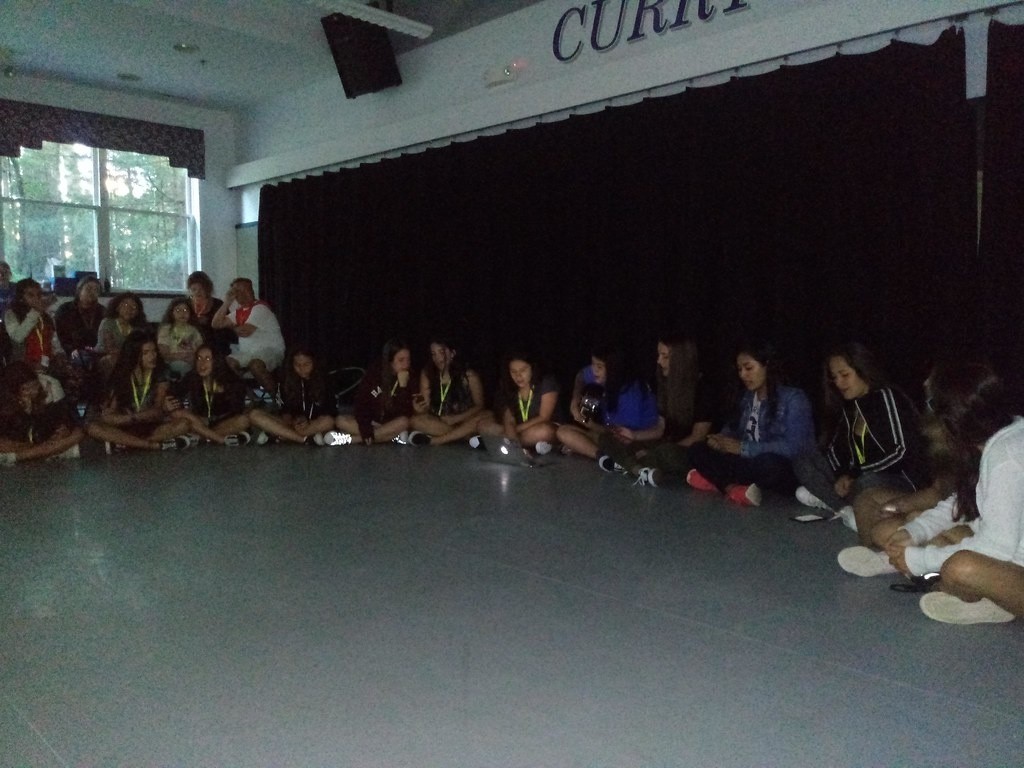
411 394 425 402
790 513 827 524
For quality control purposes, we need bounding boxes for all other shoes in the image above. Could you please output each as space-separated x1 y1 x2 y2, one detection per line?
833 507 859 532
837 545 902 577
599 455 628 474
795 486 832 510
57 443 80 459
890 573 1017 624
258 431 270 445
536 440 552 455
0 450 16 467
314 432 325 446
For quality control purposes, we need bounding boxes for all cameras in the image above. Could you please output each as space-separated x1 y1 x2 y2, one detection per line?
578 382 606 401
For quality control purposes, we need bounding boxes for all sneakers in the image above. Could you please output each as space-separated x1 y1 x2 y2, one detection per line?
224 432 250 448
186 433 205 448
105 441 125 454
687 469 718 491
409 430 430 447
162 436 190 451
391 430 408 444
324 430 352 446
636 467 675 490
728 482 762 507
470 436 485 449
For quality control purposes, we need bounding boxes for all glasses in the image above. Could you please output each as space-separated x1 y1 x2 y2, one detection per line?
121 303 138 310
197 355 214 362
174 307 190 313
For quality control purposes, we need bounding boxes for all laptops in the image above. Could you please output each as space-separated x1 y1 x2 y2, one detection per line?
479 432 559 468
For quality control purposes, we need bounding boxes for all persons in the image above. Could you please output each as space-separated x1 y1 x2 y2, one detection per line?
0 262 286 467
248 333 1024 626
